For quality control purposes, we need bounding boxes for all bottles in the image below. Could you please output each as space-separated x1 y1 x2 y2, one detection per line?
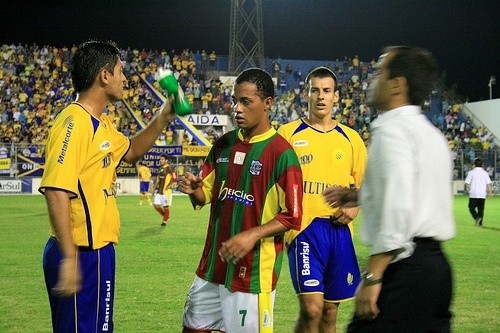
157 67 191 115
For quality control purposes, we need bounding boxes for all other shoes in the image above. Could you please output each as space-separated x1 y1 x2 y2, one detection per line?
475 217 482 225
161 221 167 225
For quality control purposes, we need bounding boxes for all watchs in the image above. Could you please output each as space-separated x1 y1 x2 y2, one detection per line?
360 271 383 286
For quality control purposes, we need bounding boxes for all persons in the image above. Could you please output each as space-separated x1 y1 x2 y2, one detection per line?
37 41 177 333
323 45 455 333
0 42 234 178
464 158 494 227
178 68 303 333
277 66 369 333
154 154 177 227
137 162 152 206
269 53 500 182
